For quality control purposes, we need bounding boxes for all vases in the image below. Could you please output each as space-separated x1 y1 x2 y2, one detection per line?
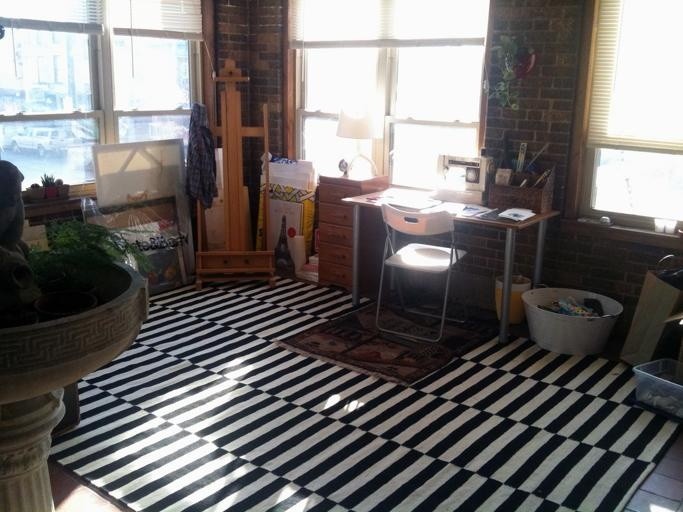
58 186 69 196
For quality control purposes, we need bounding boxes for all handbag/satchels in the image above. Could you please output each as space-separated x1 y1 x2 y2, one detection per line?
620 269 683 368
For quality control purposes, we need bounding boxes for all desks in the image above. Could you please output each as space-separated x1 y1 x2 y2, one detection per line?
340 186 563 345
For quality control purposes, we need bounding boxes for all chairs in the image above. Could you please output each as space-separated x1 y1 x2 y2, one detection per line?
375 202 467 341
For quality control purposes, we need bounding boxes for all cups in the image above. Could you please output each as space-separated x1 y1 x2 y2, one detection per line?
654 218 665 233
664 219 677 234
599 216 610 225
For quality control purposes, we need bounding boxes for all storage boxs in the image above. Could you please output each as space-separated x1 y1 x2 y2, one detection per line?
633 357 682 422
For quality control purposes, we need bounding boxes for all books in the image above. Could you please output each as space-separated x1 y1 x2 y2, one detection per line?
295 253 319 283
365 190 537 225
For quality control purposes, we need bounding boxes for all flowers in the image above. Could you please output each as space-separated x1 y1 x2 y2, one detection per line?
483 34 536 112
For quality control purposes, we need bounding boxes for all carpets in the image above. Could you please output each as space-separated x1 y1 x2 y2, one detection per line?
47 262 683 511
276 286 525 387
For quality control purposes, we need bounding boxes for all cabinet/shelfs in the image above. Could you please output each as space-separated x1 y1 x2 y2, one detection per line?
315 175 389 288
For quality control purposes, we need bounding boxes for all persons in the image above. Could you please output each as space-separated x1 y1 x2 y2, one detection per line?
0 160 26 256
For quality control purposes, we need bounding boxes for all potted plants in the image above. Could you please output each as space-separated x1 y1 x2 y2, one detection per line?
26 184 45 202
41 173 58 197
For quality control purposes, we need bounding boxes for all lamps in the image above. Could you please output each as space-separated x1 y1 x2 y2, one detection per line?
334 110 383 176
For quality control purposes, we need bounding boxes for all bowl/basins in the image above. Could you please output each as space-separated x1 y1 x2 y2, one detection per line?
521 288 624 354
